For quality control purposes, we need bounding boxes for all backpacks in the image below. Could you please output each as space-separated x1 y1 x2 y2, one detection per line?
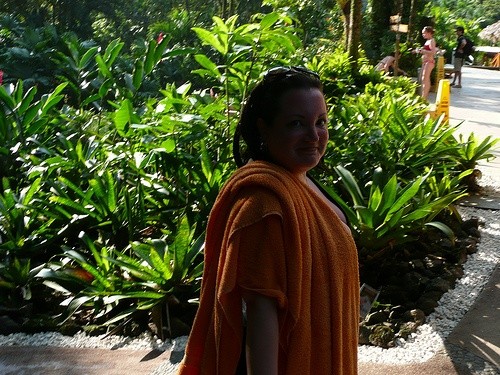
458 36 475 56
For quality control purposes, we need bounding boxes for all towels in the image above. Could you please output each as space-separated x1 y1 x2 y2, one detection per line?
175 161 360 375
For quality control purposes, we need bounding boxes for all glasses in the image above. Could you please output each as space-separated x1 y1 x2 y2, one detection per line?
247 65 319 112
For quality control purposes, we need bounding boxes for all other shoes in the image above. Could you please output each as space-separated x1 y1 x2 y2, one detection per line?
449 83 455 86
453 85 461 88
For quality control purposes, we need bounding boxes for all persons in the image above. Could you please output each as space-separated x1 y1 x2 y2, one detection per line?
370 51 410 78
449 27 467 88
410 27 438 102
179 66 358 375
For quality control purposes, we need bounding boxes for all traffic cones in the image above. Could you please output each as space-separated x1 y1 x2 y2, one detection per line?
434 56 445 94
433 79 450 126
